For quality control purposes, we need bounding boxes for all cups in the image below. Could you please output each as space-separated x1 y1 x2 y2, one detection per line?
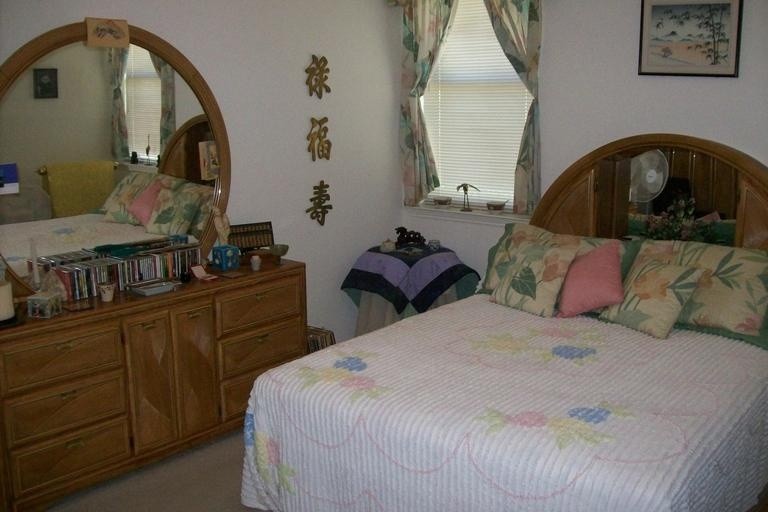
97 281 114 303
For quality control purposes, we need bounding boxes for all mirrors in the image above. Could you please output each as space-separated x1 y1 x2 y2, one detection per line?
0 20 231 298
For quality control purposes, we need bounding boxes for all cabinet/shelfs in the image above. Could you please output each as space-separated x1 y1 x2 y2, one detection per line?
118 288 222 470
215 261 311 443
0 312 133 511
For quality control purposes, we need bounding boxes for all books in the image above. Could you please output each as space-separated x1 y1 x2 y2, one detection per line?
54 242 200 302
27 233 189 290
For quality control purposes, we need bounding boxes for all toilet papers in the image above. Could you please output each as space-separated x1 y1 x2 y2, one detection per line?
0 281 14 321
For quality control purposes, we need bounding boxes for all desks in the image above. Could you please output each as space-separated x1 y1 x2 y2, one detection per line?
339 243 481 337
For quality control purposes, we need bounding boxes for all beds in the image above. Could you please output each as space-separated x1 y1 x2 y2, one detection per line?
0 111 219 279
238 130 765 512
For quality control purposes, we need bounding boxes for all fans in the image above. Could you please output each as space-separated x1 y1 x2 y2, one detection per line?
628 149 671 204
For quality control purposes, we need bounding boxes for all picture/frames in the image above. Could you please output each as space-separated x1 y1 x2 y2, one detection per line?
32 68 59 100
635 0 743 78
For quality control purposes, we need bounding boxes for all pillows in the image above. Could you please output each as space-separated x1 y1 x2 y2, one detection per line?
629 207 735 246
488 238 580 319
640 240 767 351
474 220 642 319
595 250 704 341
554 240 625 319
102 170 214 242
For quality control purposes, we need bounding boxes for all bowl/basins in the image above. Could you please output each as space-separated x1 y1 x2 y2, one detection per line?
432 195 452 210
486 201 505 215
